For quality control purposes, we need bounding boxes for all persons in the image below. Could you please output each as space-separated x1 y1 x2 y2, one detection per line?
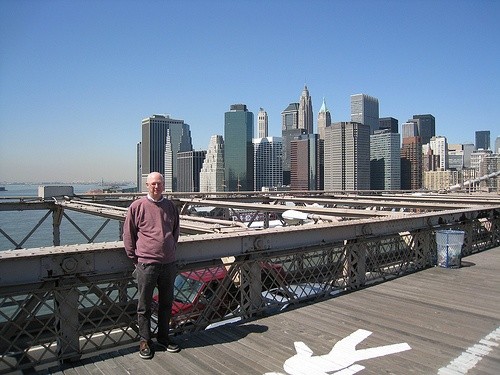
123 172 180 358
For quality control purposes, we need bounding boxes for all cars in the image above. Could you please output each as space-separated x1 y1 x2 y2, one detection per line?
143 263 288 335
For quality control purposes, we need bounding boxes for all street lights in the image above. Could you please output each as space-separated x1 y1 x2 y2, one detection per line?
281 210 339 225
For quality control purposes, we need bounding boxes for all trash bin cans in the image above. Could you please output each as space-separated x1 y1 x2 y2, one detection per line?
436 229 465 269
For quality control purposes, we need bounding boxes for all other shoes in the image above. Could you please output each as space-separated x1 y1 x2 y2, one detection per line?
151 336 180 352
138 336 153 359
